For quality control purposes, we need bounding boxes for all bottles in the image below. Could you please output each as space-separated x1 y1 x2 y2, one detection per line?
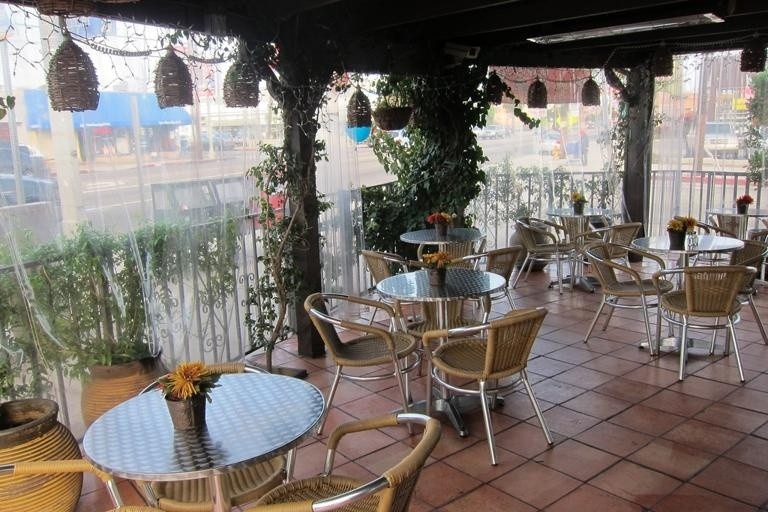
687 232 698 246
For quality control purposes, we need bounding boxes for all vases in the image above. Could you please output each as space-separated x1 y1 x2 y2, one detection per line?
737 202 748 214
574 202 583 214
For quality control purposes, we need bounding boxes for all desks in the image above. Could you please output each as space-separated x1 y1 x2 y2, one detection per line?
704 207 768 242
81 372 325 511
545 209 621 293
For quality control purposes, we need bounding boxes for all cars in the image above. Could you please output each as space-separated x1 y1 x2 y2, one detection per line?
0 137 58 203
535 131 565 156
683 120 741 161
180 124 234 152
469 120 512 140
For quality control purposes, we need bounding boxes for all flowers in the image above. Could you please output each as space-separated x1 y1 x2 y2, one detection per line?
570 192 589 205
426 211 458 225
737 194 753 204
